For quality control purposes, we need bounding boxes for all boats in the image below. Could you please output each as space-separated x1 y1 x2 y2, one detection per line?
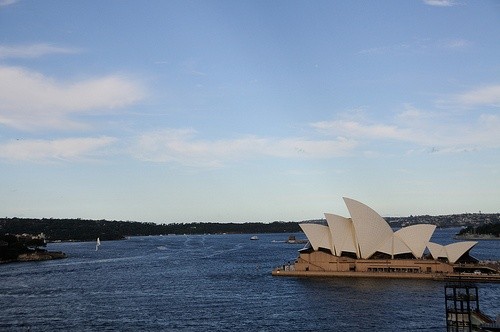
251 236 259 240
